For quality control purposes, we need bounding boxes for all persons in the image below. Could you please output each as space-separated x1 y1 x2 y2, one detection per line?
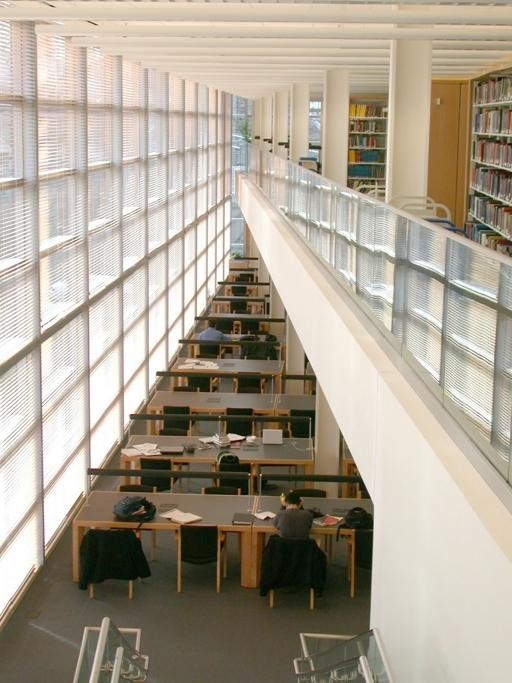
199 319 223 340
272 492 313 539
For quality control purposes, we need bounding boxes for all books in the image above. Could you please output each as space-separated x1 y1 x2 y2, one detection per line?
199 433 246 449
347 104 388 194
232 512 254 524
303 514 344 520
178 358 219 370
464 75 512 258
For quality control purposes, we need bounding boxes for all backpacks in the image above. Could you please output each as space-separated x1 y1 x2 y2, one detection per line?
344 506 372 528
112 495 156 523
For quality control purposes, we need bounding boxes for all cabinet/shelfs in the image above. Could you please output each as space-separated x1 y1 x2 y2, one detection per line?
463 67 512 258
347 94 388 194
